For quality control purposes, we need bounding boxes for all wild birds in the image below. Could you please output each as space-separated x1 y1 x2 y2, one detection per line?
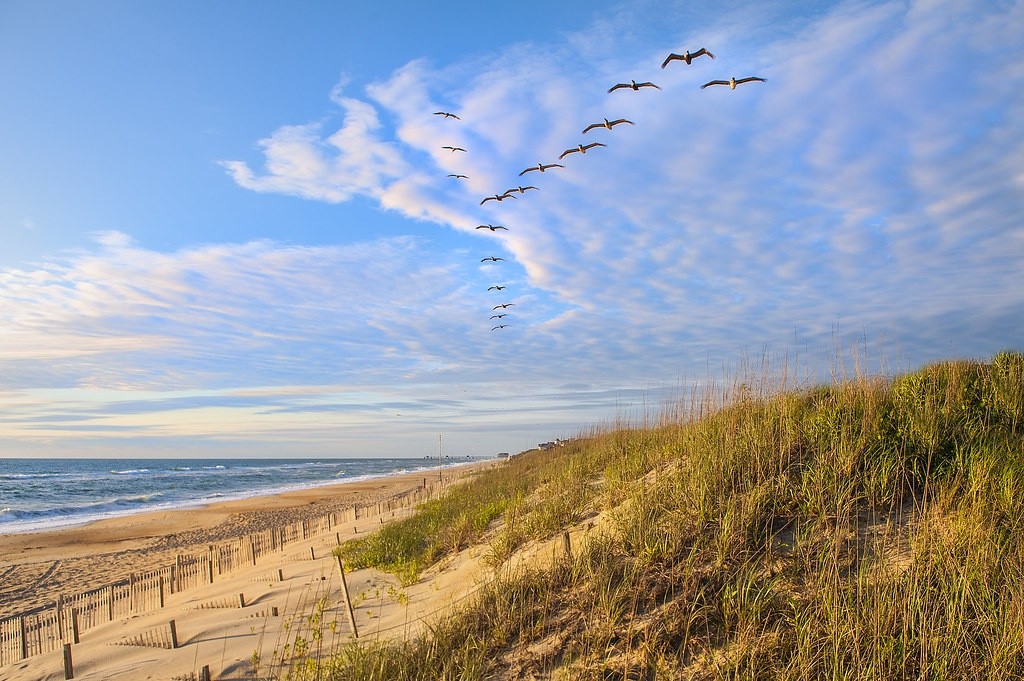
476 225 508 231
504 186 540 195
433 112 460 120
699 77 768 90
481 257 507 262
607 80 663 94
581 118 636 134
519 163 566 176
491 325 509 330
559 142 606 160
479 195 516 205
661 46 715 69
447 174 469 179
442 147 467 153
493 304 515 309
488 286 506 291
490 314 509 319
